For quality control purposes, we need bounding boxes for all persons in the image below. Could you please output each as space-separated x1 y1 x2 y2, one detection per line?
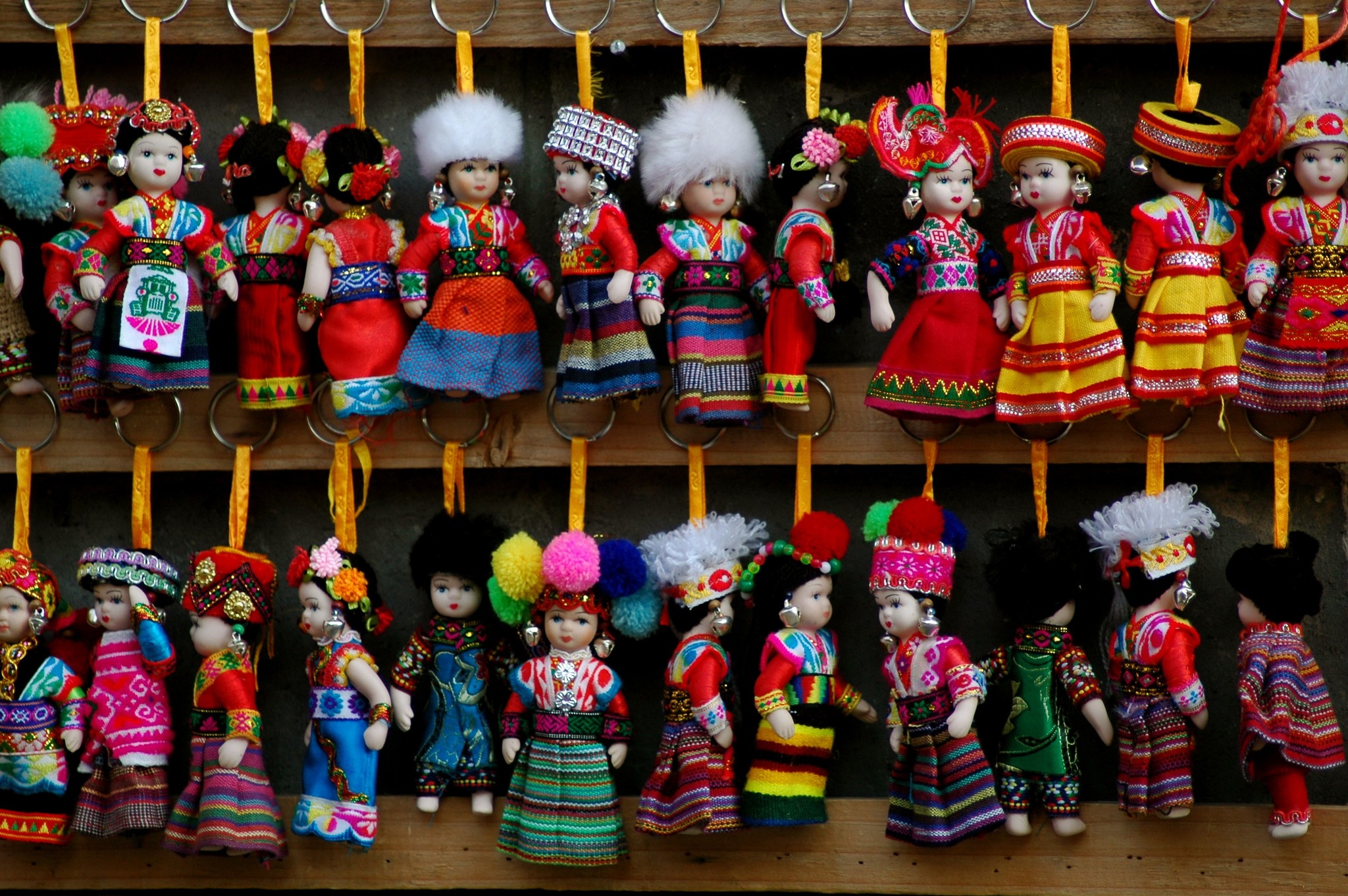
0 59 1347 425
0 484 1346 868
